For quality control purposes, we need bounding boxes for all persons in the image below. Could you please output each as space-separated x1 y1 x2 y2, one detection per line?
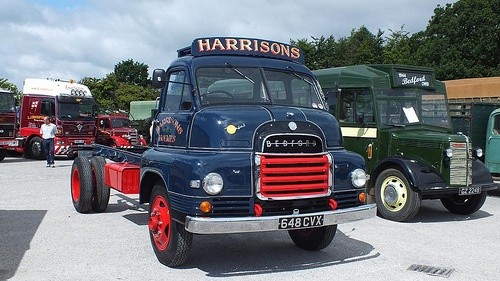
40 116 57 167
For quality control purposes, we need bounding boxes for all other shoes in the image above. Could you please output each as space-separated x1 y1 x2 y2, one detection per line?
51 164 55 166
46 163 50 166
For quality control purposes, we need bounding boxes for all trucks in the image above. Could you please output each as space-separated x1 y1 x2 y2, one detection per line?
0 77 99 163
94 109 149 149
420 76 500 182
128 100 160 144
205 63 500 223
67 36 378 267
